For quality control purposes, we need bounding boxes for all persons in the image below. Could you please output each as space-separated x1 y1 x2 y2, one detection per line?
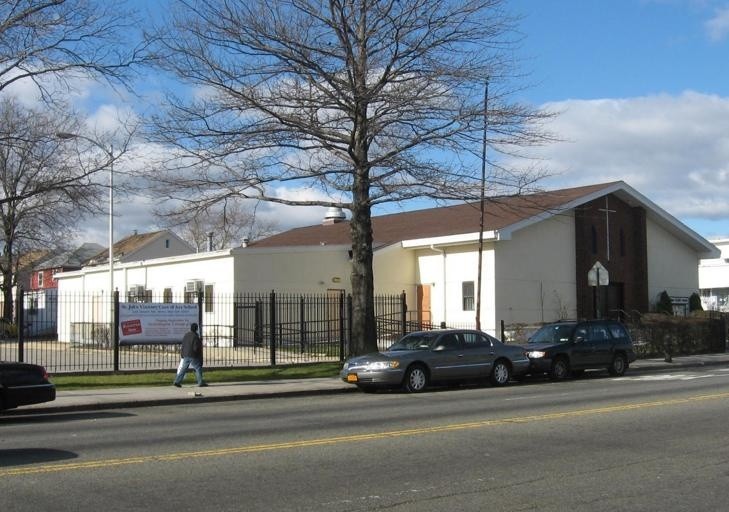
172 322 209 387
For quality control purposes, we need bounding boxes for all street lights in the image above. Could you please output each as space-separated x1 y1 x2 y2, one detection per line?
56 132 115 293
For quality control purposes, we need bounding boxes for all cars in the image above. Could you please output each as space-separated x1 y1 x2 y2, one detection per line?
0 362 56 412
340 319 638 393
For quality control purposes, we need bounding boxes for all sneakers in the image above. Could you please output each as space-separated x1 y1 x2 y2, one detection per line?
198 384 208 387
173 383 182 387
